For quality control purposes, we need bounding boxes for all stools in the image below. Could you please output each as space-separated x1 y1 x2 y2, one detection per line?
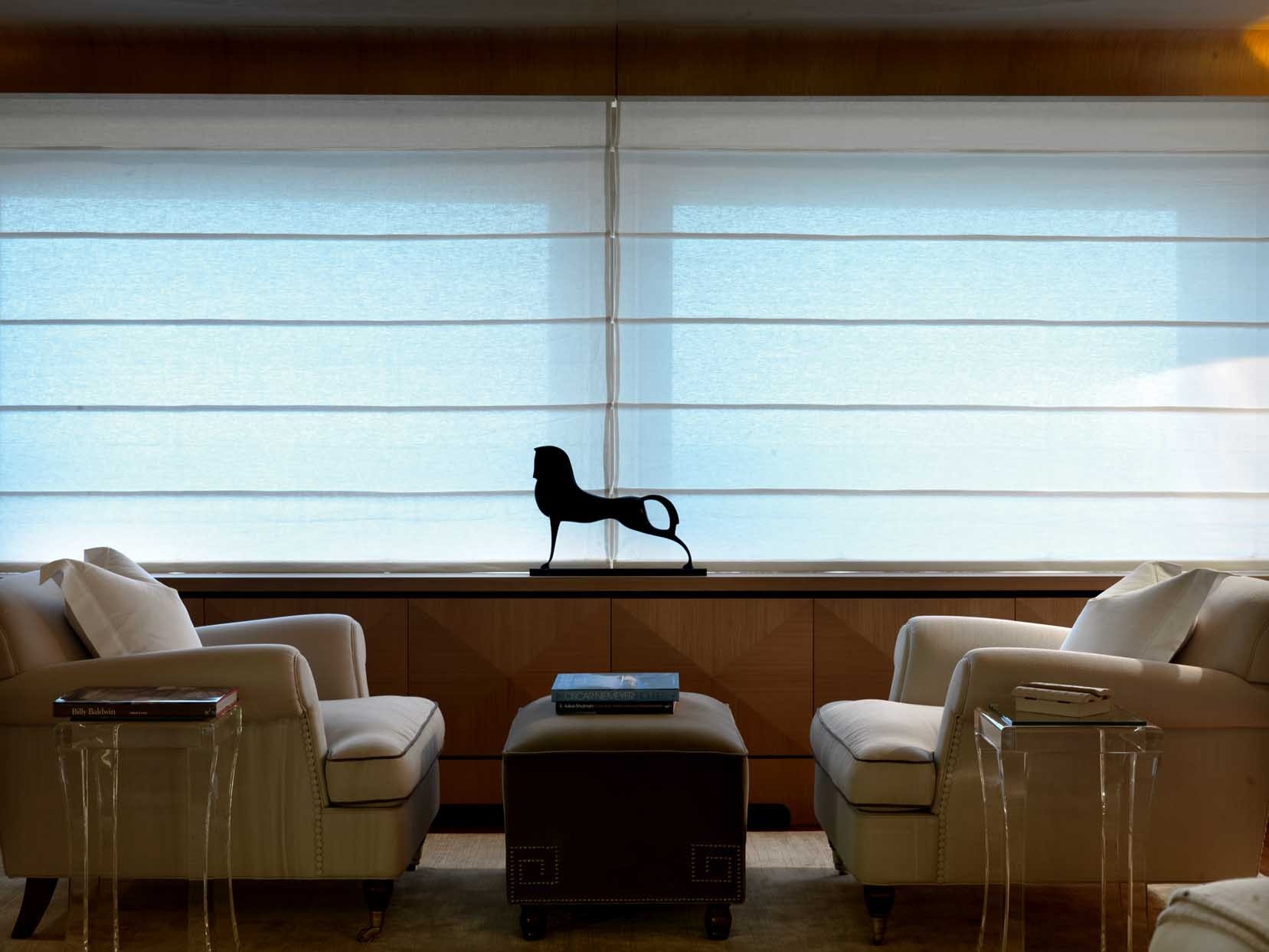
502 693 751 945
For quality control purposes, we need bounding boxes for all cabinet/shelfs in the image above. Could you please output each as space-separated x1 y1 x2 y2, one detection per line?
183 577 1115 832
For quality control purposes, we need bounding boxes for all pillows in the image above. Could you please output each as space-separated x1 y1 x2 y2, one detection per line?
39 547 203 660
1061 560 1238 663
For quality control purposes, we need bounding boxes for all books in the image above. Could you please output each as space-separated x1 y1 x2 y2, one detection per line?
1011 680 1116 717
52 685 239 721
551 670 680 715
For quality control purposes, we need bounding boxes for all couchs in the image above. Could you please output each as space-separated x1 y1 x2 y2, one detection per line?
809 577 1269 952
0 567 446 940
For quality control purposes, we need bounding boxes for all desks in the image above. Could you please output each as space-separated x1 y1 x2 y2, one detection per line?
56 705 242 951
974 703 1166 952
